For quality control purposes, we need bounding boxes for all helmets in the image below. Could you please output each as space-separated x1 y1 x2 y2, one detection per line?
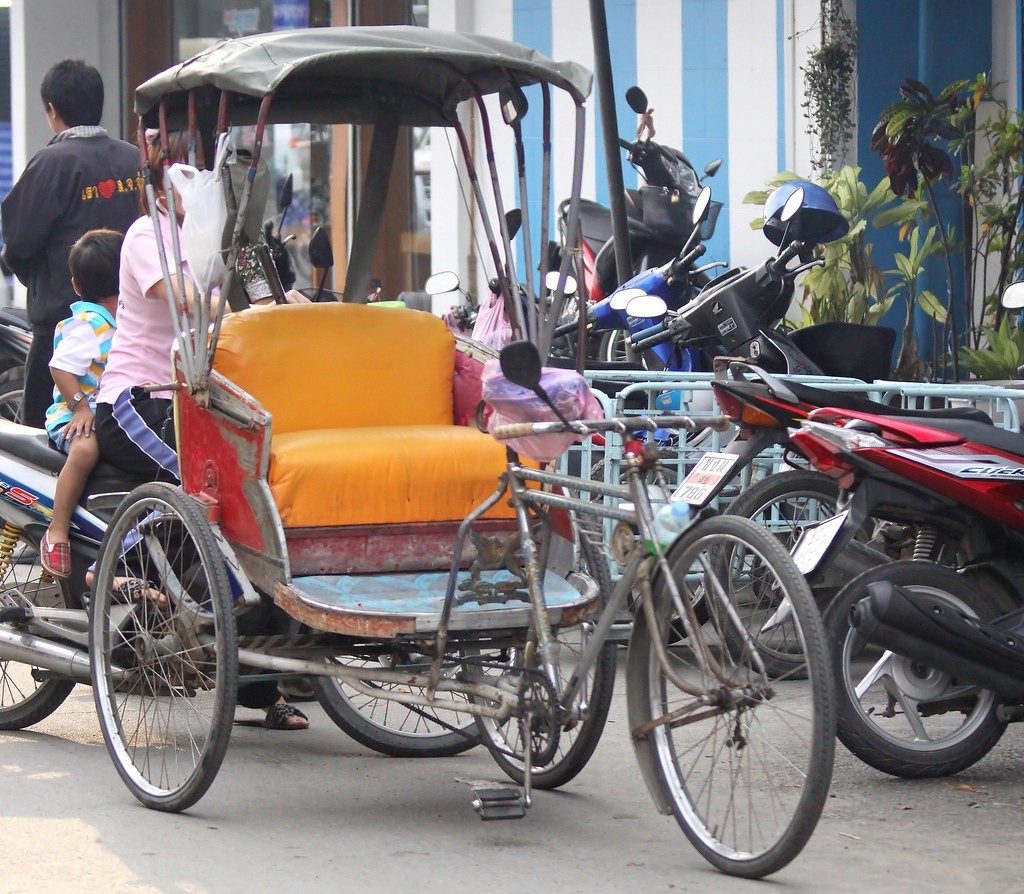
763 180 850 247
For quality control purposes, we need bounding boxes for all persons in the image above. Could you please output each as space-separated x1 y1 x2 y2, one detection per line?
85 131 315 728
0 60 144 565
40 229 126 577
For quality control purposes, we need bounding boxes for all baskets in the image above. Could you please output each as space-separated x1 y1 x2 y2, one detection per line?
640 186 724 240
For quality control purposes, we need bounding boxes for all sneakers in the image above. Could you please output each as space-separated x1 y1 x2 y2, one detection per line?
40 529 72 579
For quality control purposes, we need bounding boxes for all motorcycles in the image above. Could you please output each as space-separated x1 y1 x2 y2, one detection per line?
622 179 896 591
546 186 729 443
539 84 724 300
0 173 297 427
0 227 524 759
787 406 1024 779
672 361 994 681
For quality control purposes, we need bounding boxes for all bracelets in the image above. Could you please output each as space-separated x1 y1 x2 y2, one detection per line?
67 391 86 412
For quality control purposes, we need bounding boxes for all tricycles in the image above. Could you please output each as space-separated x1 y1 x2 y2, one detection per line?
90 27 839 879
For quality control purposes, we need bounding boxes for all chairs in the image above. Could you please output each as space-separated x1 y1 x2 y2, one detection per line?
208 302 542 576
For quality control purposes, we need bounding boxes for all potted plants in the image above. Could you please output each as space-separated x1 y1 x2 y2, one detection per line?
744 69 1023 428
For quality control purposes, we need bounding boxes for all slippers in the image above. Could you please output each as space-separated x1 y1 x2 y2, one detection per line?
232 698 310 730
80 576 168 608
